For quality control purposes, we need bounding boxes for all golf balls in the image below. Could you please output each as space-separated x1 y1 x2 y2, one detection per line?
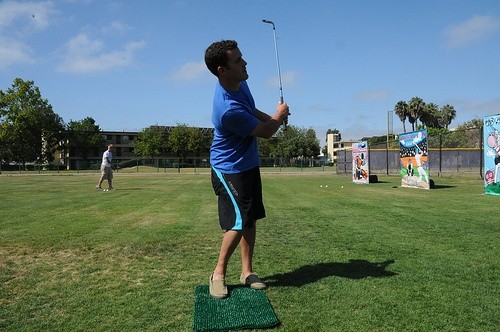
320 184 343 188
103 189 108 191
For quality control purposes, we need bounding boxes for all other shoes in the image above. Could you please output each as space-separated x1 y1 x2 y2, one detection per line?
240 274 266 289
209 271 228 298
96 185 102 189
108 186 114 189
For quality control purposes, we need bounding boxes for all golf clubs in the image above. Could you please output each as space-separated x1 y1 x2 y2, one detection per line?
262 19 287 131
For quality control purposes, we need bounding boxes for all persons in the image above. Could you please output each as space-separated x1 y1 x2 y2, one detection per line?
96 144 115 190
204 39 291 298
